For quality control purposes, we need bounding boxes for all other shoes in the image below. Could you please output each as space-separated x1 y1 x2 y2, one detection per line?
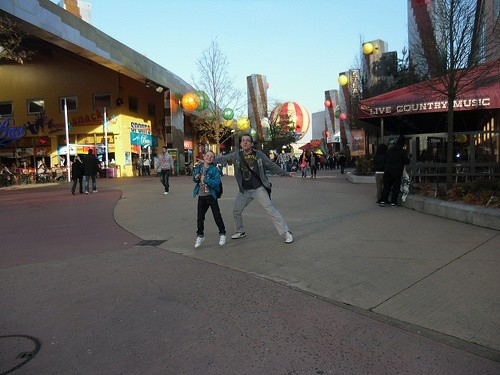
379 201 387 206
85 191 89 194
79 191 84 194
71 190 76 195
92 190 98 193
390 202 397 207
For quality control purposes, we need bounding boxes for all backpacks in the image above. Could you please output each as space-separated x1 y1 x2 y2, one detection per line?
217 179 223 199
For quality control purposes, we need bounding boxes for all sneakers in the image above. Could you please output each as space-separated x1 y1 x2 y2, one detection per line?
163 192 168 195
284 231 294 243
231 232 247 238
194 236 205 248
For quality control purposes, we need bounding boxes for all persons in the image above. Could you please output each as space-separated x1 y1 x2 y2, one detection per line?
299 149 309 178
155 146 174 195
380 135 410 207
372 144 389 204
188 152 223 176
192 150 226 248
71 155 84 195
197 134 297 243
276 148 357 171
0 162 64 187
339 153 347 174
132 154 153 177
83 149 101 194
307 151 320 178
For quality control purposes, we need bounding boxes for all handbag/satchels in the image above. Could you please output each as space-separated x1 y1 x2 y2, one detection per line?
219 236 226 246
265 182 272 200
